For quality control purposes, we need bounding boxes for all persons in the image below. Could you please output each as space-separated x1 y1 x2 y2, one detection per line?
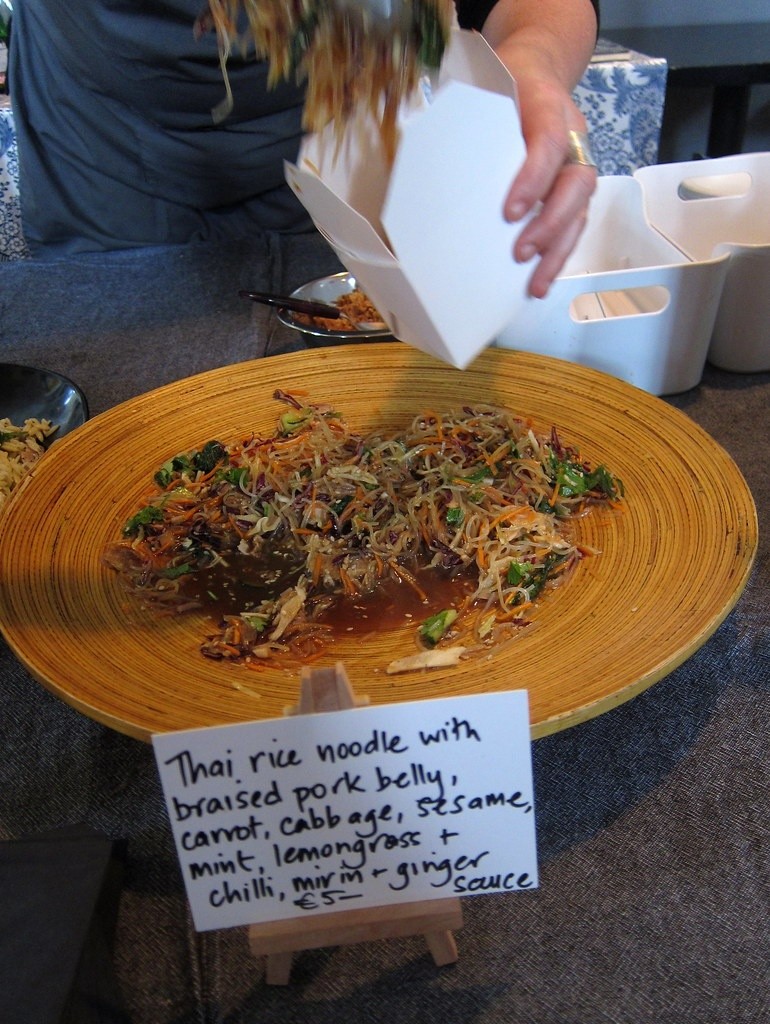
9 0 600 299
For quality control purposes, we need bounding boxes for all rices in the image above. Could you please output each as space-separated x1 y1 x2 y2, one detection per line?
0 418 60 506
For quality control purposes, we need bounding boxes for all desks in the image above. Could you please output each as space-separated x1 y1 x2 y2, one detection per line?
0 232 770 1024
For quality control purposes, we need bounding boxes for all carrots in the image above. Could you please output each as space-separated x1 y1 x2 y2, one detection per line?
295 288 385 329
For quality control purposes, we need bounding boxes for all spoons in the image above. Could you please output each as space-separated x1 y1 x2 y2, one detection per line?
236 289 389 331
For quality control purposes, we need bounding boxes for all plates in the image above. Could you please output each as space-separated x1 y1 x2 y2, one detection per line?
0 338 757 753
276 272 398 348
0 359 89 518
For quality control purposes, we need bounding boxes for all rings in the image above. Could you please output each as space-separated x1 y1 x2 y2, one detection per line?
563 130 597 167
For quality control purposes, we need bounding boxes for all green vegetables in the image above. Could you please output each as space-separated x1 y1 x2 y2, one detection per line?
125 408 624 644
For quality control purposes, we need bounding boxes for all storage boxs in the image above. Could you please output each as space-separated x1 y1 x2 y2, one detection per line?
262 28 770 400
